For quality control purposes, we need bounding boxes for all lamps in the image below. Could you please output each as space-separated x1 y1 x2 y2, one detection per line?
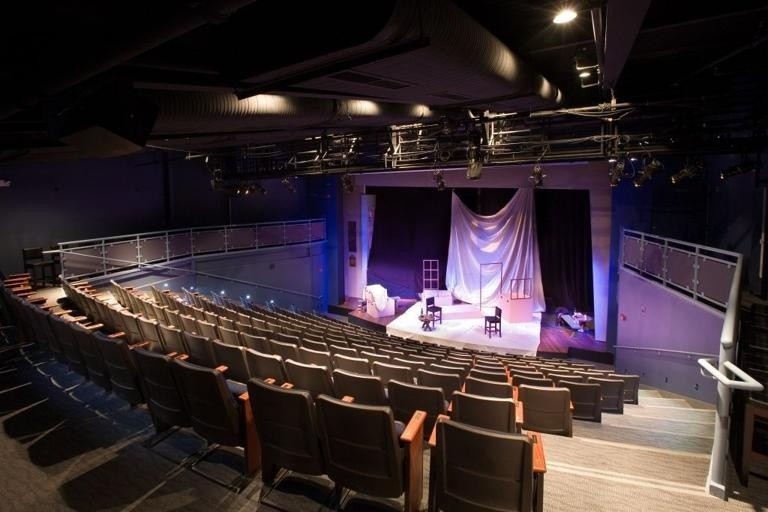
602 139 752 193
208 171 299 196
335 146 552 195
